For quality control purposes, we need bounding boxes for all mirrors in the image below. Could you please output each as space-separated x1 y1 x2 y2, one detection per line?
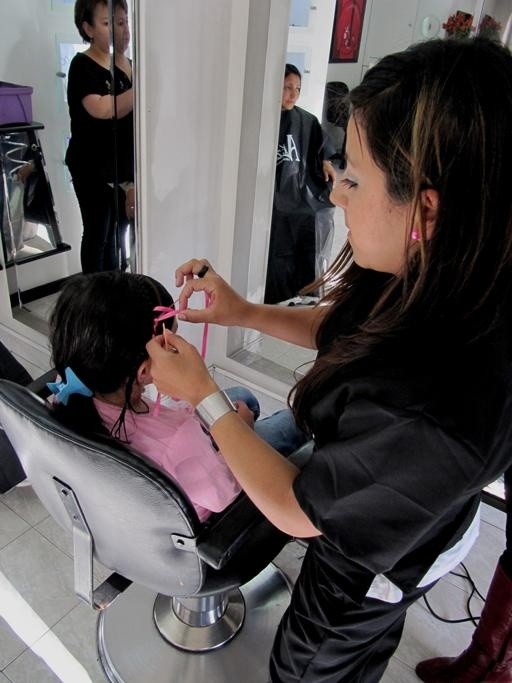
0 0 146 355
219 1 511 393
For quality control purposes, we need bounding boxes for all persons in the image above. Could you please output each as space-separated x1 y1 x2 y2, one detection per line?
258 56 337 307
140 33 512 682
39 269 324 521
64 0 116 275
114 0 137 272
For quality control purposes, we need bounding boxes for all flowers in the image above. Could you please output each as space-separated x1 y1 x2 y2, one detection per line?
439 11 477 39
477 13 501 38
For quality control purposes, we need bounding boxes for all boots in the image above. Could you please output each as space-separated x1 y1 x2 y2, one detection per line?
413 549 511 683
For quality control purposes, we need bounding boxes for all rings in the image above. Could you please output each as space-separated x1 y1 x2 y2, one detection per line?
196 263 210 279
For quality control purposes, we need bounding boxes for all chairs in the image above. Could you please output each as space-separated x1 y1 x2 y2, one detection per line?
1 376 316 680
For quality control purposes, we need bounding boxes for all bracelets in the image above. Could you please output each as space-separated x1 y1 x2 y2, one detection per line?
193 386 237 432
